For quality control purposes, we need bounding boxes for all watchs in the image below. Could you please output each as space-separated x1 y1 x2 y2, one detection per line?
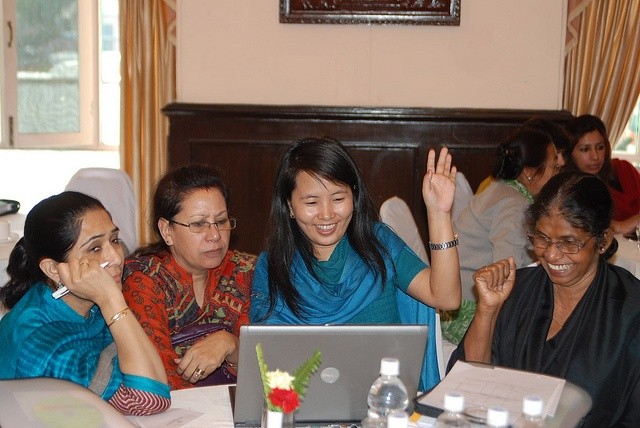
429 234 458 250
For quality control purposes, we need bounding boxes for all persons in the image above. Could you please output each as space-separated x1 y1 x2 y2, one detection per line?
566 115 640 237
1 191 171 414
121 164 257 391
249 137 462 393
473 117 567 195
438 170 640 427
440 129 560 343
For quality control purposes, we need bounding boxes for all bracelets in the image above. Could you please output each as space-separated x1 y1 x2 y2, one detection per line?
107 307 130 327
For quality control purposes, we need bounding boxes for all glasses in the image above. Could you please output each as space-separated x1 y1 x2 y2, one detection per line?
538 162 562 174
167 218 236 231
526 228 603 254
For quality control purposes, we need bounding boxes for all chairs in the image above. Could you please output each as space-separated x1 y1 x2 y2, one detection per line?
65 167 139 255
451 171 473 224
379 196 457 381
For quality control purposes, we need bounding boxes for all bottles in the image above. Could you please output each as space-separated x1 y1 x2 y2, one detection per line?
521 396 545 427
364 358 410 427
486 407 512 427
434 393 472 428
385 411 408 427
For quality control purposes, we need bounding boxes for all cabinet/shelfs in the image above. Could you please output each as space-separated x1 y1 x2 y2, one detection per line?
162 101 576 258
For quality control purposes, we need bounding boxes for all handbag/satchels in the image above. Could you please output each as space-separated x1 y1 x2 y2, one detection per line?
170 323 238 385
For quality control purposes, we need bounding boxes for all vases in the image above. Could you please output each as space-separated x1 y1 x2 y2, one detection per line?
261 409 295 428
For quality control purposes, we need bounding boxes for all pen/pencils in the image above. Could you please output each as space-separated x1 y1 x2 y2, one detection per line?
52 261 110 299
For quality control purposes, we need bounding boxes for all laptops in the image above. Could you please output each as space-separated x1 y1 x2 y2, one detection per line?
228 325 427 424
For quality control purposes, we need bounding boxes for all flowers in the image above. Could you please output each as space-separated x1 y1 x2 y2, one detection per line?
255 343 323 414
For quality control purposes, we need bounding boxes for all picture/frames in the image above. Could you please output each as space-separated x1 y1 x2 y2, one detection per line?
277 2 460 27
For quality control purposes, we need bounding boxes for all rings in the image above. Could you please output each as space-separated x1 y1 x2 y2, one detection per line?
486 265 488 271
194 367 204 377
